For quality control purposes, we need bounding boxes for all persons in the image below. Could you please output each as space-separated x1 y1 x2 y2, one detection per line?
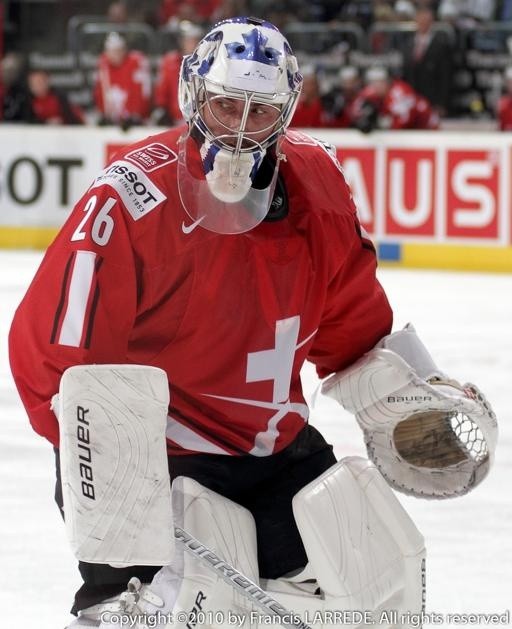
7 16 499 629
0 0 512 131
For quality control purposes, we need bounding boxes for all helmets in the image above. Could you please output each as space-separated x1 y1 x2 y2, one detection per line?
178 16 304 203
